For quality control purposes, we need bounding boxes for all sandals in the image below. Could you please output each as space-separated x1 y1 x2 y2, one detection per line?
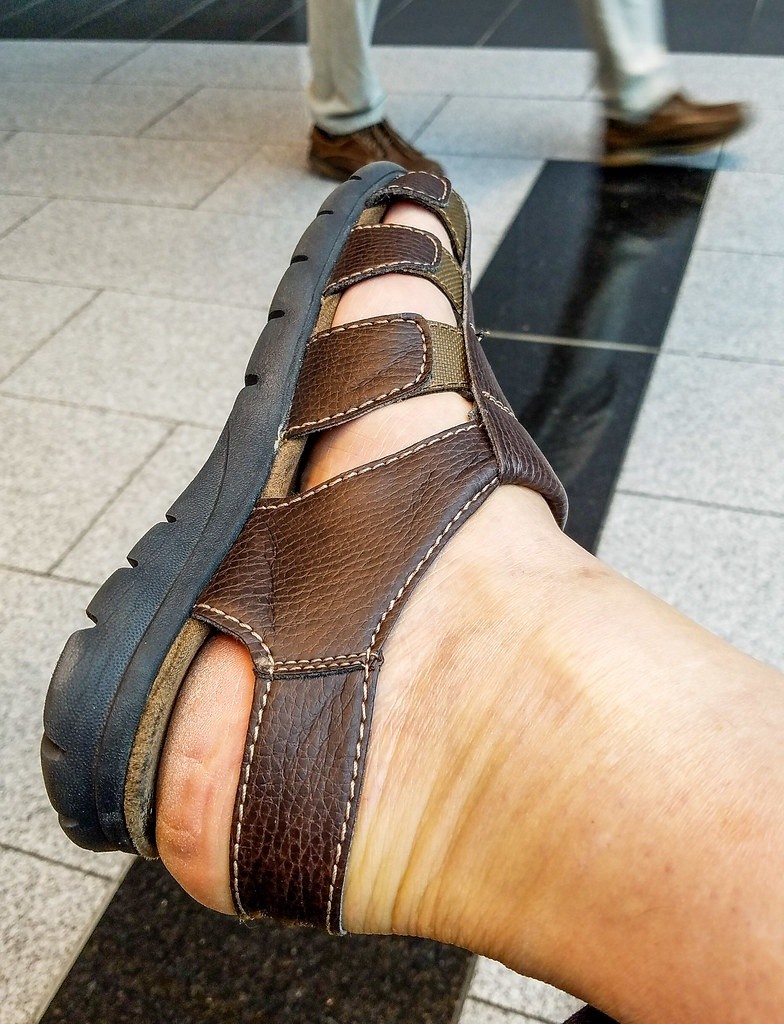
39 168 575 938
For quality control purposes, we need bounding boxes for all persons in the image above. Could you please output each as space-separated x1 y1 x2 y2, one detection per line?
41 161 784 1024
306 0 753 181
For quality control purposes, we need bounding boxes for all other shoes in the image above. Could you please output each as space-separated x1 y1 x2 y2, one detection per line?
604 88 742 156
306 122 446 181
